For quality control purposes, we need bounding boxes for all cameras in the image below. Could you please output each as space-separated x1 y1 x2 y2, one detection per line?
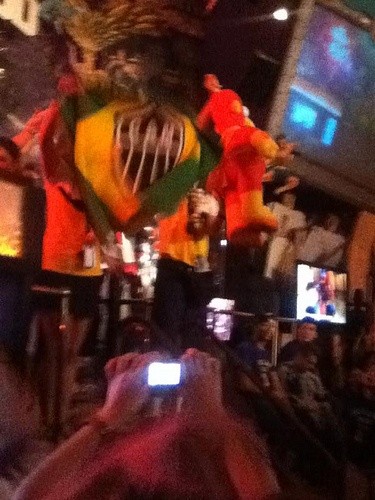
140 359 188 390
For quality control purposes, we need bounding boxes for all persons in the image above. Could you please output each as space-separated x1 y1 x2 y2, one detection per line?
0 72 375 499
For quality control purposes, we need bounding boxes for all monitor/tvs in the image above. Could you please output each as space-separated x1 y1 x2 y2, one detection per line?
291 257 351 330
